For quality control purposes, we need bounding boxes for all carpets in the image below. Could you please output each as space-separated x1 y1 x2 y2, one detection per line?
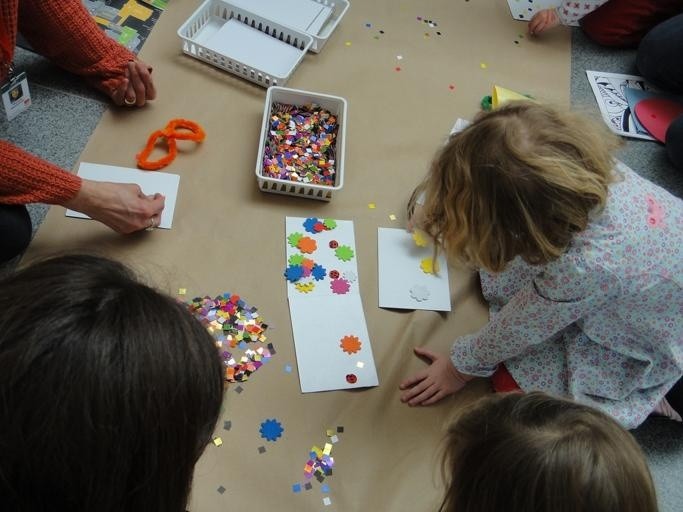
0 0 572 510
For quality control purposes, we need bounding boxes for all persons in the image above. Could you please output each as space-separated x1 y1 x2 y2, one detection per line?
438 392 657 512
0 0 165 261
636 14 683 171
528 0 683 46
0 255 223 512
399 102 683 430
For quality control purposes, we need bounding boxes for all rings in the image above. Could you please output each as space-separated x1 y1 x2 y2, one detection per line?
145 218 154 231
125 99 136 104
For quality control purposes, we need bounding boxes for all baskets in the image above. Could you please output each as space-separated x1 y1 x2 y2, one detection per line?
255 85 347 201
175 1 351 88
